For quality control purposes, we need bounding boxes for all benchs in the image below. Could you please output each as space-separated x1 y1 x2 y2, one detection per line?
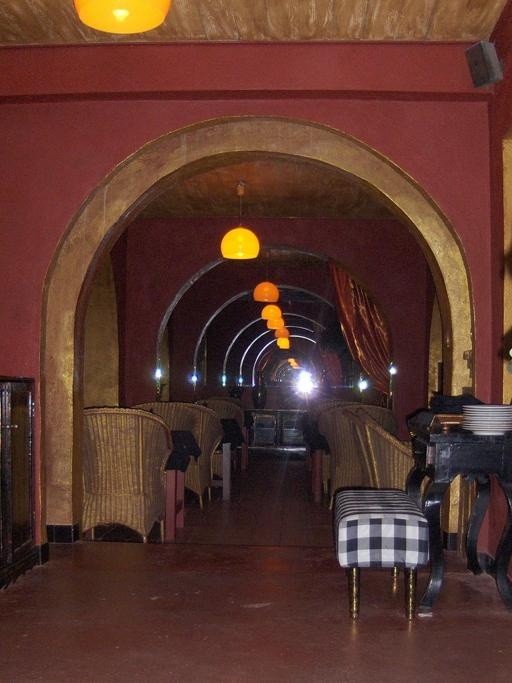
333 488 431 619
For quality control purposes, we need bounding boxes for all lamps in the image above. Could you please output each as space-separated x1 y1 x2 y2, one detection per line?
76 0 171 35
253 259 300 369
220 180 260 260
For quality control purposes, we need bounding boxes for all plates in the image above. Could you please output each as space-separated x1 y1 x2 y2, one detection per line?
425 390 486 413
460 402 512 437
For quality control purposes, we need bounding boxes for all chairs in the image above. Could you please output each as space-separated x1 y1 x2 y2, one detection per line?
132 403 225 509
195 399 245 471
81 408 174 543
311 400 414 509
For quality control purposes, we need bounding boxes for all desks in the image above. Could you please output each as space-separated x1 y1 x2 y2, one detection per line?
245 409 308 451
406 409 512 617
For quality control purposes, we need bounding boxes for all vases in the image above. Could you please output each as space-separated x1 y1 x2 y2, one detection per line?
252 370 266 408
